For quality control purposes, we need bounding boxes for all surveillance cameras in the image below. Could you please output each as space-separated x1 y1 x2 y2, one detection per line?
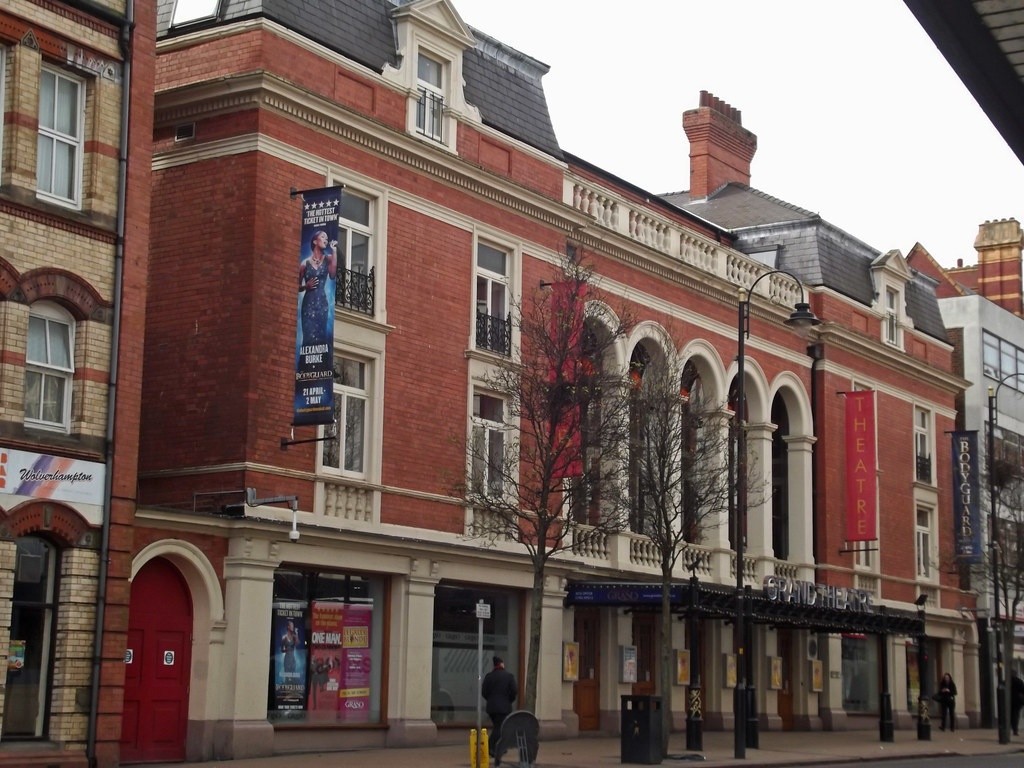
986 628 993 633
289 530 300 543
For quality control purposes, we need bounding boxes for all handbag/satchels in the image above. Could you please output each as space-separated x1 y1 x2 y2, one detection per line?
933 691 953 701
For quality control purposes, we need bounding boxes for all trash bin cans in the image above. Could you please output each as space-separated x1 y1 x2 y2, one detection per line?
618 693 664 765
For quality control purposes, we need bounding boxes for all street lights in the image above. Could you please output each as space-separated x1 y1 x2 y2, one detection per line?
735 268 823 760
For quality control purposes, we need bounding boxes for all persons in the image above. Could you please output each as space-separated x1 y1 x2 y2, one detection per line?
281 620 299 683
1003 668 1024 736
481 656 517 759
937 673 957 731
298 231 338 345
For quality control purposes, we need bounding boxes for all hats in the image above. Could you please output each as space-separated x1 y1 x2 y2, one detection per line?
493 657 503 666
